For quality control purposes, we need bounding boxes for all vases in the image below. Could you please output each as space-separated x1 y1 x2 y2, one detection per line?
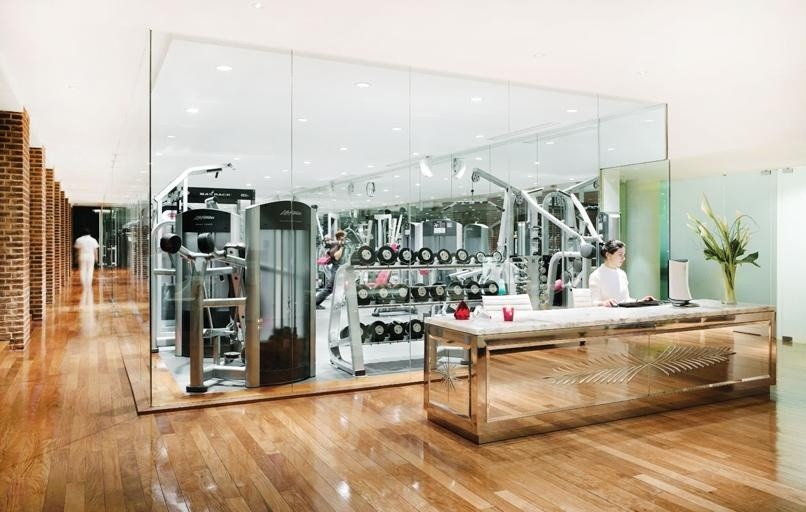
721 265 736 307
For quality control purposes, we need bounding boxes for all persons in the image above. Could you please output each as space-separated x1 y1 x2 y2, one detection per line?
316 232 345 309
589 240 655 307
74 228 100 287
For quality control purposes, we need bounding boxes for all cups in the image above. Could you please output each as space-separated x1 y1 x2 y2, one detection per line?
502 306 515 323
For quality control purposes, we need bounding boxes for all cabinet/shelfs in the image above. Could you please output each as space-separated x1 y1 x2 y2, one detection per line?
326 261 519 373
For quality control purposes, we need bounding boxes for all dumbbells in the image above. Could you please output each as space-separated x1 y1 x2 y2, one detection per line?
519 261 548 309
340 246 502 341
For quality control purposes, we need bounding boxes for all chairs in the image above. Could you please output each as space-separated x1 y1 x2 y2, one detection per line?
565 285 591 309
479 289 533 315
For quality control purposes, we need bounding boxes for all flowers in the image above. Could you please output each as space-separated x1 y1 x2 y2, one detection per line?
685 189 763 302
